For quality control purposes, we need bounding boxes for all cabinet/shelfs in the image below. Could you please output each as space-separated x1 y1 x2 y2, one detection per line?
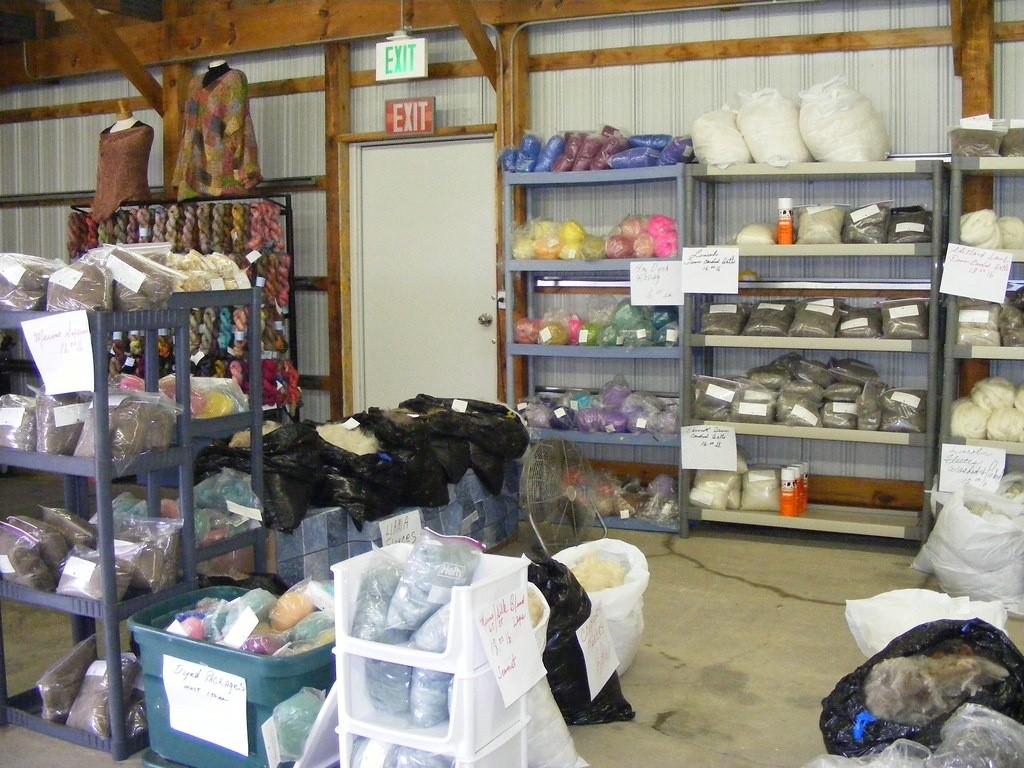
505 154 1024 539
0 289 265 760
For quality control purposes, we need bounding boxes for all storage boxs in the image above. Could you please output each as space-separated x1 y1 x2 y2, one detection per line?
328 543 531 768
128 583 337 768
948 128 1003 157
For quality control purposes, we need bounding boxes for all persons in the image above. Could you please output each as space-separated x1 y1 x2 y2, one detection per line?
89 99 155 223
171 60 264 203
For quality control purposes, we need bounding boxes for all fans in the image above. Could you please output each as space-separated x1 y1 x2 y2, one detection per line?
517 436 607 559
